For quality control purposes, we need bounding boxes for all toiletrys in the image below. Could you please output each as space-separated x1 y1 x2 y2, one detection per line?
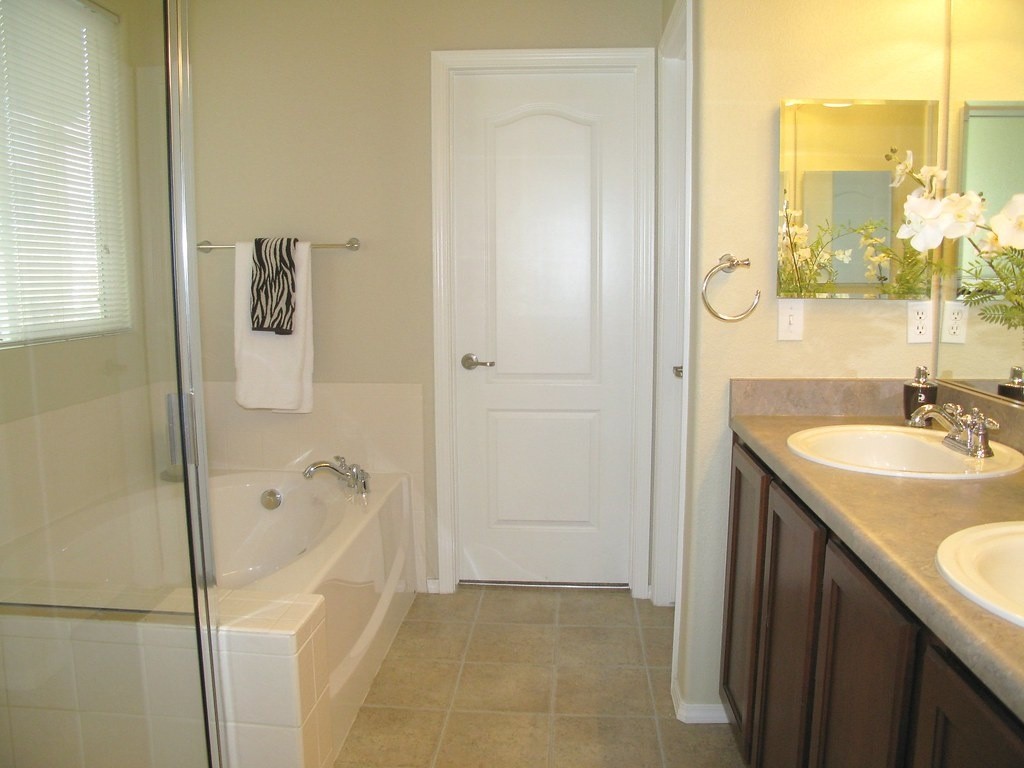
902 365 938 428
997 365 1024 401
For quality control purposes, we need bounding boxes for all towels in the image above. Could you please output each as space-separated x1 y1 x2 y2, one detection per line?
251 237 299 336
234 240 312 414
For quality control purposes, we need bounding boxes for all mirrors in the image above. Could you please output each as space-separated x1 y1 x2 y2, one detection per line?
933 0 1024 410
776 98 937 303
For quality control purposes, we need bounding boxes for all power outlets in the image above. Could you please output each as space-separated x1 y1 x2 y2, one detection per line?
906 302 932 344
940 301 968 344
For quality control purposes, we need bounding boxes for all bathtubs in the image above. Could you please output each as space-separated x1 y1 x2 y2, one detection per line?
0 460 411 768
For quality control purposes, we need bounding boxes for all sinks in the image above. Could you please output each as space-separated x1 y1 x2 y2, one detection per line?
787 424 1022 478
935 520 1024 626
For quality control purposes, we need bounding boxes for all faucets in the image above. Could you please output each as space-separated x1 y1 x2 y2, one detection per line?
303 461 349 479
910 405 960 436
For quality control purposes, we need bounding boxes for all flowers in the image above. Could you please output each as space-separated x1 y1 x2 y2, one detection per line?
778 199 931 303
882 143 1024 329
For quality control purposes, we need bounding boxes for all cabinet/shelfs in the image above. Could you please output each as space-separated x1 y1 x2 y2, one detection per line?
717 432 1024 768
